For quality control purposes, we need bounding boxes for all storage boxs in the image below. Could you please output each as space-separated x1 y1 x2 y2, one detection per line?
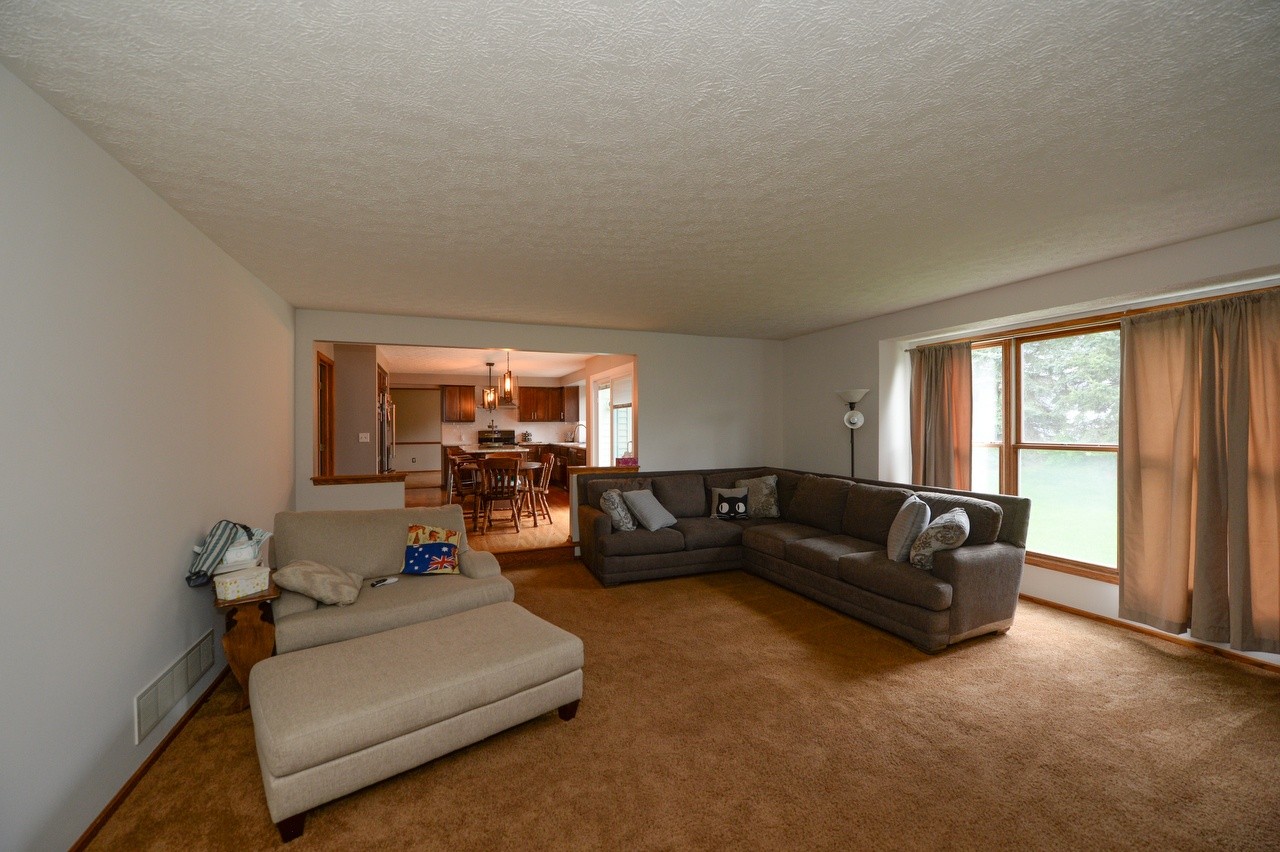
214 568 273 602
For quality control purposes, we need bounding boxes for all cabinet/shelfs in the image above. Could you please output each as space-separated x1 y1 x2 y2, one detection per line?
521 445 587 491
442 384 476 423
515 387 579 424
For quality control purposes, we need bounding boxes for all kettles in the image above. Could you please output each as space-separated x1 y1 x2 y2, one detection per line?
565 432 573 441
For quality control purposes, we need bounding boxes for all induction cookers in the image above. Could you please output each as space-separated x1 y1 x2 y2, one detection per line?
478 430 518 447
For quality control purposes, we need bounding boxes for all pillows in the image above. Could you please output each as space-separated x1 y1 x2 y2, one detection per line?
400 522 462 578
274 558 367 607
733 472 780 517
886 493 933 561
710 485 750 520
910 504 971 570
622 489 678 532
599 488 637 533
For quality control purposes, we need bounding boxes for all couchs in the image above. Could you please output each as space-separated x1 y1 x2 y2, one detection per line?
267 507 515 654
577 466 1034 659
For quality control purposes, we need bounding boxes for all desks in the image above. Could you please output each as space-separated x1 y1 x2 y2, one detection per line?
461 458 542 528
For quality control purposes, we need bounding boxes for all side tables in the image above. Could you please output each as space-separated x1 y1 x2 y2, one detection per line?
213 565 280 714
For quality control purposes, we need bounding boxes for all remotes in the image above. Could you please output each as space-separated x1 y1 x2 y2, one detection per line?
370 577 398 588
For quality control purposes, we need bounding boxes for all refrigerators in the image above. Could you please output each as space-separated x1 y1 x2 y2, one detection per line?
376 393 396 474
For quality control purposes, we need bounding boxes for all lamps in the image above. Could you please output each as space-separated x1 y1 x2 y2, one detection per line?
480 361 498 413
503 350 513 403
834 386 872 478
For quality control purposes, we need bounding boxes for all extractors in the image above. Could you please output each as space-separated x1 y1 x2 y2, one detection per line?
478 396 519 409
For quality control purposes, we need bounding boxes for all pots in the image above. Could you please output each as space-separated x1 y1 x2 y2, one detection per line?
521 431 532 442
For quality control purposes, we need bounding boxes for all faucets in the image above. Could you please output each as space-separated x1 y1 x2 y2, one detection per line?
574 424 586 431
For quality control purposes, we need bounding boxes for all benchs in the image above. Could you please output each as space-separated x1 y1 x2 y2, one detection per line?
247 598 587 844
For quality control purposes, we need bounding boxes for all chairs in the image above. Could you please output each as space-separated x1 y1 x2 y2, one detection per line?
444 448 558 539
